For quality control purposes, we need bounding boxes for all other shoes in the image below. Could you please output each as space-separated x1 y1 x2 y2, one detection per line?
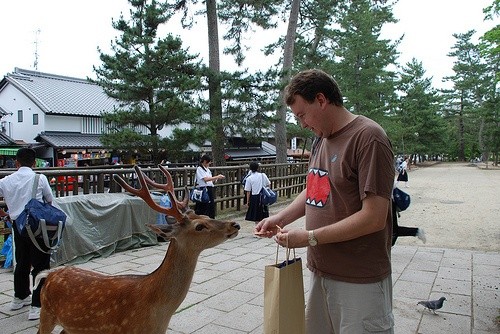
253 234 263 239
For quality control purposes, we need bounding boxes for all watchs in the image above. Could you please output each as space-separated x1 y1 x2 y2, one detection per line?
308 230 317 247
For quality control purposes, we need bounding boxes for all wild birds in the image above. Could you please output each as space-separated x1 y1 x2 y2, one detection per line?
417 297 447 316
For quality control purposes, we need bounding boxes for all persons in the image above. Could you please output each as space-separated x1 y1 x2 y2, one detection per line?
255 70 395 334
242 160 271 239
0 148 54 321
397 157 410 188
190 155 225 219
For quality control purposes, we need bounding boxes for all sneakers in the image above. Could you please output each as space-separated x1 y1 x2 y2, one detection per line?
9 295 32 309
28 305 42 319
415 227 426 244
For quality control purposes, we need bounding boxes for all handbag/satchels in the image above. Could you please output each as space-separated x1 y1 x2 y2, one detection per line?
263 233 305 334
190 187 209 204
14 173 67 254
259 186 277 205
393 187 410 212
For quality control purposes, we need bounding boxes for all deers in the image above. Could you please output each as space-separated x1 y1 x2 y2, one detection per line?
36 163 241 334
